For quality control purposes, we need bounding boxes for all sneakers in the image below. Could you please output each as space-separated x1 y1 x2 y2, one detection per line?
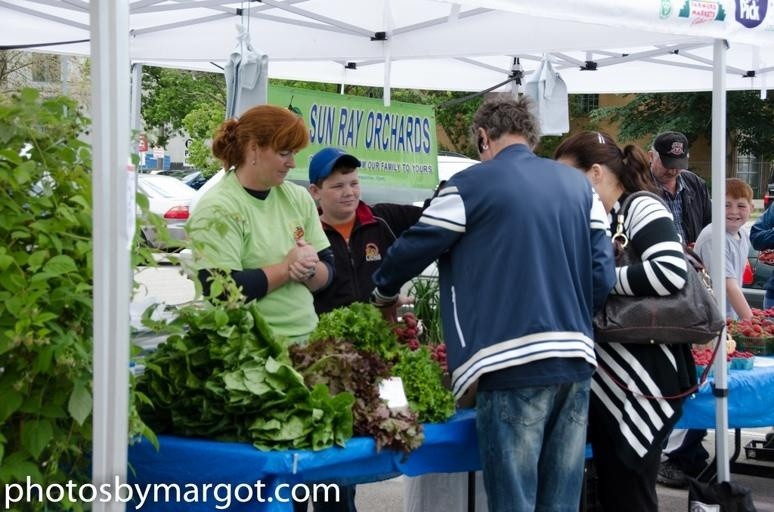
655 461 689 489
763 432 774 448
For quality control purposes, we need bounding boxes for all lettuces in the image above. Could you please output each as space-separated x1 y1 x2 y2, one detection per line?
304 302 457 425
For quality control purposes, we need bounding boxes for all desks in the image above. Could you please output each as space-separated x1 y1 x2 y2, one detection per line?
125 352 774 511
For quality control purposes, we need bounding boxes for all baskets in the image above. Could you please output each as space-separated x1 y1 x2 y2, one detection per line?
697 337 773 378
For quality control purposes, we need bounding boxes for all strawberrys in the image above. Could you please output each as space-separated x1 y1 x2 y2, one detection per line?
726 305 774 338
692 346 751 365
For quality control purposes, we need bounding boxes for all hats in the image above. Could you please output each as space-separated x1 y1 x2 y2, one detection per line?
654 131 689 170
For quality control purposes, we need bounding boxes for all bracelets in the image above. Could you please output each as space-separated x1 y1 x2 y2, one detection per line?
309 266 317 280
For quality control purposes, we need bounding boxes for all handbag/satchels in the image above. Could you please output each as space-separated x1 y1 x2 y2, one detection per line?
592 190 724 400
688 477 757 512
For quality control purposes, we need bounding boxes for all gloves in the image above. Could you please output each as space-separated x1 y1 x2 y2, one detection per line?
308 147 361 182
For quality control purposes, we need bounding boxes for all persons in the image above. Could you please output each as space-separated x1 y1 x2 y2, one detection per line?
749 199 774 310
647 130 712 246
368 95 617 512
308 147 398 317
185 102 341 349
654 176 754 488
554 129 700 510
